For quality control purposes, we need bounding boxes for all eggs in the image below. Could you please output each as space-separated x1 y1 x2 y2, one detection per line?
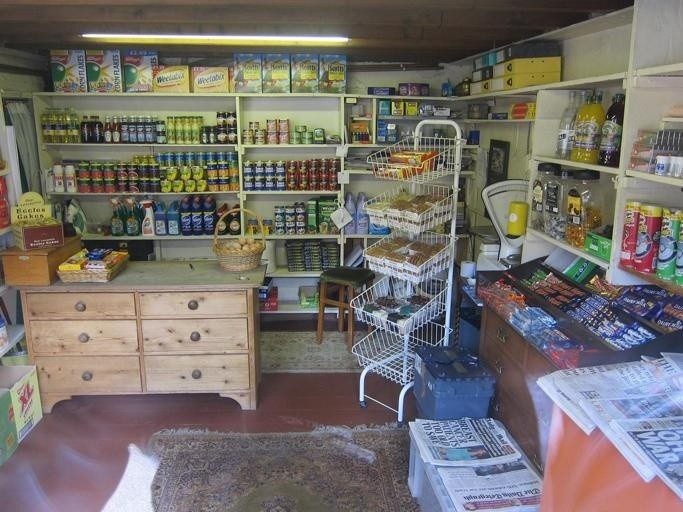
218 237 263 249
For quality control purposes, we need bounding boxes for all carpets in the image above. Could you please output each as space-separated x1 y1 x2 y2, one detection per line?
258 331 393 375
144 422 432 511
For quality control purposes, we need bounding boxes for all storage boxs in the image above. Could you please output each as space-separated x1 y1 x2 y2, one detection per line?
232 51 262 94
119 48 159 93
318 54 346 94
472 66 493 82
472 51 496 72
261 51 291 93
289 52 318 92
489 72 561 92
406 420 546 512
152 63 190 93
85 48 126 93
190 66 230 94
469 79 491 96
0 388 17 469
493 56 561 79
413 345 496 422
495 39 561 65
0 365 44 444
1 235 82 288
49 49 88 93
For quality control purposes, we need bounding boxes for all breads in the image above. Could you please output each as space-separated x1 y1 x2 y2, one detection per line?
367 236 446 267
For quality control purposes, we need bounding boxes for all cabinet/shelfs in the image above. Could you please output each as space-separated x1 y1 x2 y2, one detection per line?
478 301 569 480
33 92 242 263
134 290 258 410
451 0 683 304
343 63 468 301
238 93 343 324
541 354 683 511
350 119 463 426
0 90 27 360
21 290 145 415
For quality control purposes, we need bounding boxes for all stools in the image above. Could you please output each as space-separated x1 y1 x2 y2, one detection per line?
315 265 374 354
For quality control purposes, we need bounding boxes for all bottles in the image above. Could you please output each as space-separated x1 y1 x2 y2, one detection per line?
578 87 605 164
120 116 156 145
597 93 625 168
555 91 575 158
529 163 604 249
180 194 215 236
104 117 120 143
167 115 205 146
40 106 79 143
570 96 590 162
564 115 575 161
79 115 103 144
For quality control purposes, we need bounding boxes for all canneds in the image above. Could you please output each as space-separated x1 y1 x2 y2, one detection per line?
117 152 206 193
287 158 340 190
200 126 216 144
206 151 240 192
243 122 267 144
155 121 166 144
267 120 290 145
290 125 313 145
216 111 237 144
242 160 286 190
274 205 306 236
78 162 117 193
263 220 273 237
248 220 258 237
